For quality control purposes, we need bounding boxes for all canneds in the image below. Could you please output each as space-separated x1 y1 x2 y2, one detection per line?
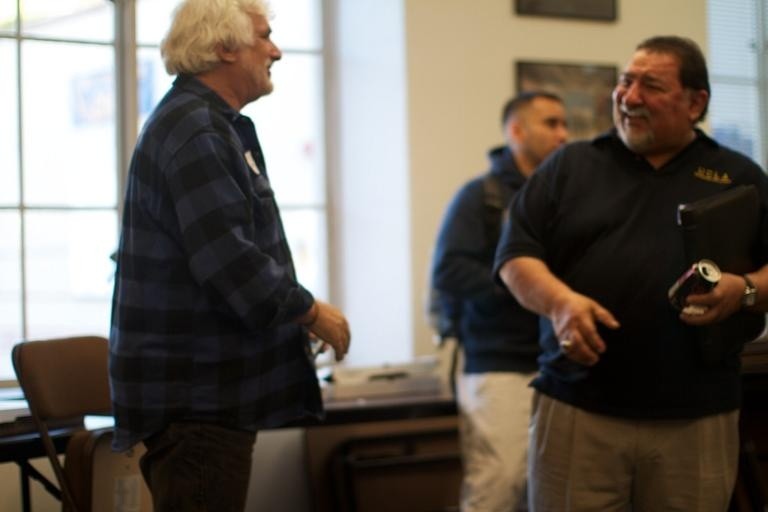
668 258 722 309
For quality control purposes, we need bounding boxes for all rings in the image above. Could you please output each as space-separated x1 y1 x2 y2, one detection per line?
561 339 574 353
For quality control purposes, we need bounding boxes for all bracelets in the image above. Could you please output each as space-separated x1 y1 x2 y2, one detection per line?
301 299 320 327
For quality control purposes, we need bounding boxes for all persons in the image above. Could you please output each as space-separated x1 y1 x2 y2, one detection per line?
103 0 352 510
426 89 576 512
489 33 766 512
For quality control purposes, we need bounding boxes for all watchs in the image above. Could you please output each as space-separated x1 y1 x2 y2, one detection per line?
737 276 757 310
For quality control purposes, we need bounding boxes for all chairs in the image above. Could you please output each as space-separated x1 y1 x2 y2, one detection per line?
11 337 154 512
